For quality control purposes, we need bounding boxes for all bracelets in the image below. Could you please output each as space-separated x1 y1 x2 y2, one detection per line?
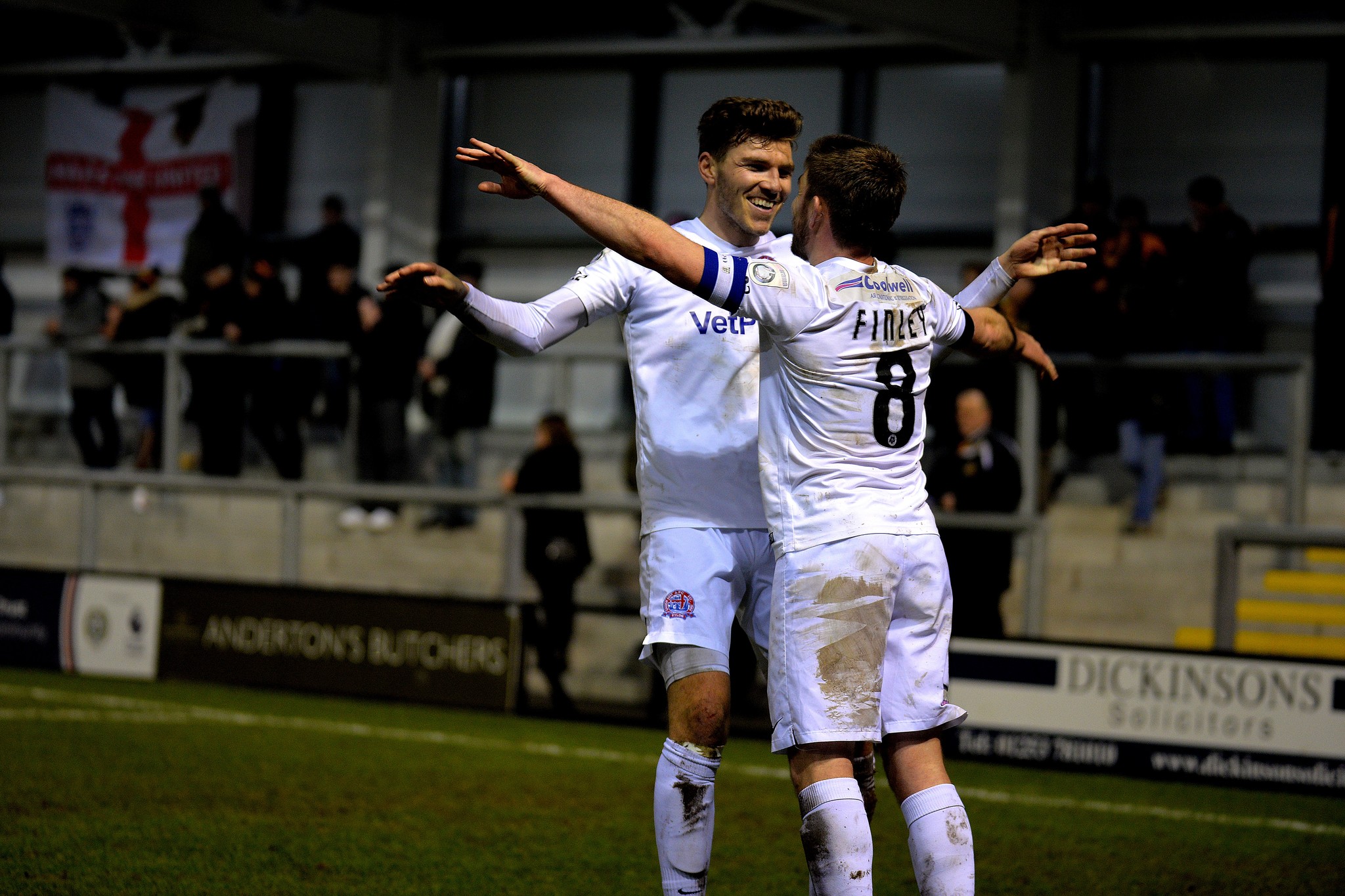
1001 314 1017 353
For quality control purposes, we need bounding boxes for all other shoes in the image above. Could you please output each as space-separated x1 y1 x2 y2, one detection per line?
1122 515 1150 535
340 506 367 530
1104 459 1124 503
368 506 393 532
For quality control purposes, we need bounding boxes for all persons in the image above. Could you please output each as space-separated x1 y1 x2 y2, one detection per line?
457 136 1056 896
1 186 598 712
378 97 1098 896
929 156 1345 633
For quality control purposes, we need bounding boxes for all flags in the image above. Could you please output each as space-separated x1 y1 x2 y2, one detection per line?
34 81 256 272
